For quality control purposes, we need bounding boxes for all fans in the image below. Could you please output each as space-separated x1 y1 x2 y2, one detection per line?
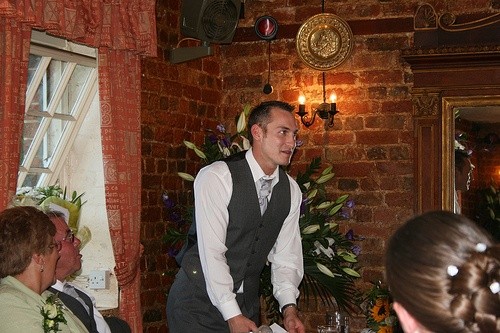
165 0 242 64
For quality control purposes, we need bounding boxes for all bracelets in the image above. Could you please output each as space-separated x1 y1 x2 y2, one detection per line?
282 303 298 313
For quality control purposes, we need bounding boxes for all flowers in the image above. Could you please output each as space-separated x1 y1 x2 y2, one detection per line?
358 280 398 333
141 103 364 333
38 292 68 333
13 184 91 251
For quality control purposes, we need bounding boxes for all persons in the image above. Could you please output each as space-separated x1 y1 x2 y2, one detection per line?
385 210 500 333
165 100 306 333
453 149 474 215
46 211 112 333
0 207 89 333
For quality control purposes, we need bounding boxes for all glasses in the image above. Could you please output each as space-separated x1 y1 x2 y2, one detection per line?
58 233 74 243
49 240 62 251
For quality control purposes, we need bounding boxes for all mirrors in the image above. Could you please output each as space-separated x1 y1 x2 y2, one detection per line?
440 95 500 244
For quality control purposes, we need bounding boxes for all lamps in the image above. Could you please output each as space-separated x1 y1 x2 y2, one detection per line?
296 92 339 128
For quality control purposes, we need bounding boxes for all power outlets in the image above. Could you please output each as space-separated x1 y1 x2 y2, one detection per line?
89 269 109 289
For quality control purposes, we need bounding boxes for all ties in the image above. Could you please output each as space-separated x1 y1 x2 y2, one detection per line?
64 286 89 315
258 179 271 215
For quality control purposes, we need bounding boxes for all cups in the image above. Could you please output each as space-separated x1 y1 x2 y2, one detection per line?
317 325 341 333
